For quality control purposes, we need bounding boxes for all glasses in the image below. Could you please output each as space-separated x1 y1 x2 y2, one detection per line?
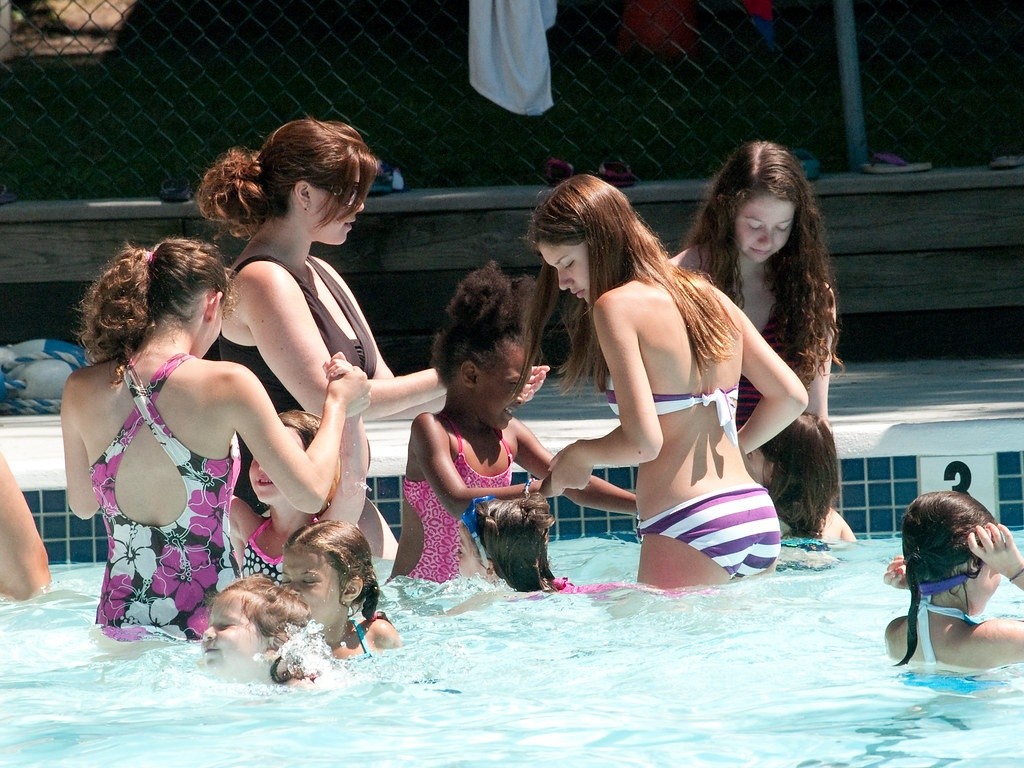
461 494 496 570
287 178 361 207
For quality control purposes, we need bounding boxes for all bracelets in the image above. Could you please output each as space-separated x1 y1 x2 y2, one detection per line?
1009 568 1024 582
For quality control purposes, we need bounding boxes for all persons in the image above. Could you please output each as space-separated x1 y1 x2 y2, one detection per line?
513 174 808 590
200 577 319 685
883 490 1024 668
672 141 844 431
61 240 371 643
458 492 574 592
748 412 856 541
0 452 51 602
387 260 637 584
229 352 368 590
196 120 550 560
281 520 402 658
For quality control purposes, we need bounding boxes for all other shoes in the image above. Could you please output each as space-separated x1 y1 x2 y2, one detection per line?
589 161 636 186
369 164 410 193
158 177 192 200
545 158 575 187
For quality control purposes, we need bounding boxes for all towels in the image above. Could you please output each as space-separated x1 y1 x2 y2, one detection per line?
467 0 559 118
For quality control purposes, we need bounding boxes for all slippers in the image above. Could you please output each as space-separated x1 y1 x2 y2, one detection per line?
989 145 1024 168
859 151 932 173
